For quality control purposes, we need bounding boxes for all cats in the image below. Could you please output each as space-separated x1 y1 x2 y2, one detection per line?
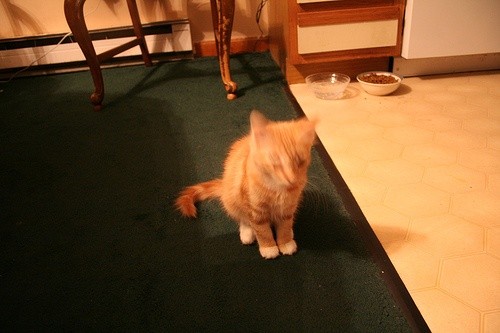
173 108 321 260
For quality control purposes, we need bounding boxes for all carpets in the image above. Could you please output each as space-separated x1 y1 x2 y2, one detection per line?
0 51 432 333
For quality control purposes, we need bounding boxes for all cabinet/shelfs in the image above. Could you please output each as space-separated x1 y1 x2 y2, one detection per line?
264 0 408 85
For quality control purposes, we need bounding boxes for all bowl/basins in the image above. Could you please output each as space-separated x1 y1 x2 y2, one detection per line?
356 71 402 96
305 72 350 99
